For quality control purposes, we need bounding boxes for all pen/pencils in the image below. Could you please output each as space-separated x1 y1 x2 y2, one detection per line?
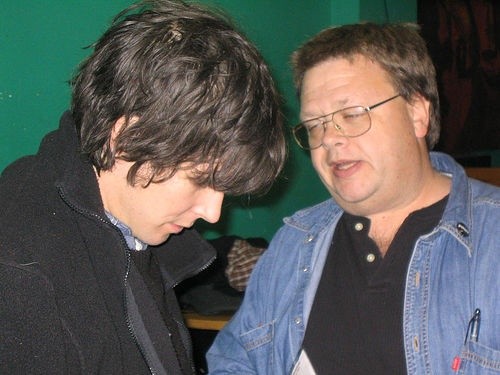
463 308 481 345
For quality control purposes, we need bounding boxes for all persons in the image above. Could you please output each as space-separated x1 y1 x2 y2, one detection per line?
0 0 286 375
206 22 500 375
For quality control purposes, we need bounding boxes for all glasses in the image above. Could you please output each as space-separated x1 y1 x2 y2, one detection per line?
290 93 401 150
